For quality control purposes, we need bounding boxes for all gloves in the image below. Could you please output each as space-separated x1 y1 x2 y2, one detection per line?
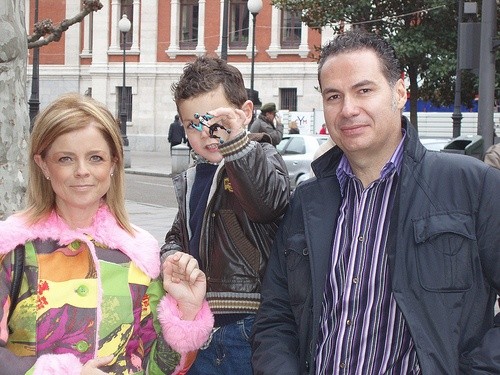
169 138 171 142
183 139 187 144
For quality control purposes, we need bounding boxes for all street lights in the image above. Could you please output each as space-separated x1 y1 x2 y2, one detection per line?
247 0 263 101
118 14 132 147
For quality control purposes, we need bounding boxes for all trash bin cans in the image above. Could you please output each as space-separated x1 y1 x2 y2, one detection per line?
440 133 484 160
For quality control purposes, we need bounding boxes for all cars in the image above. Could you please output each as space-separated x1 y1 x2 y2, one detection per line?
275 135 328 193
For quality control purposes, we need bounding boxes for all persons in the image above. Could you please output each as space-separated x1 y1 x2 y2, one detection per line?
159 57 292 375
167 103 328 154
252 33 500 375
0 91 213 375
188 113 231 145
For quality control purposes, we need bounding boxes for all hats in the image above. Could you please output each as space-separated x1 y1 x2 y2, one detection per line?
175 115 179 119
260 103 278 112
